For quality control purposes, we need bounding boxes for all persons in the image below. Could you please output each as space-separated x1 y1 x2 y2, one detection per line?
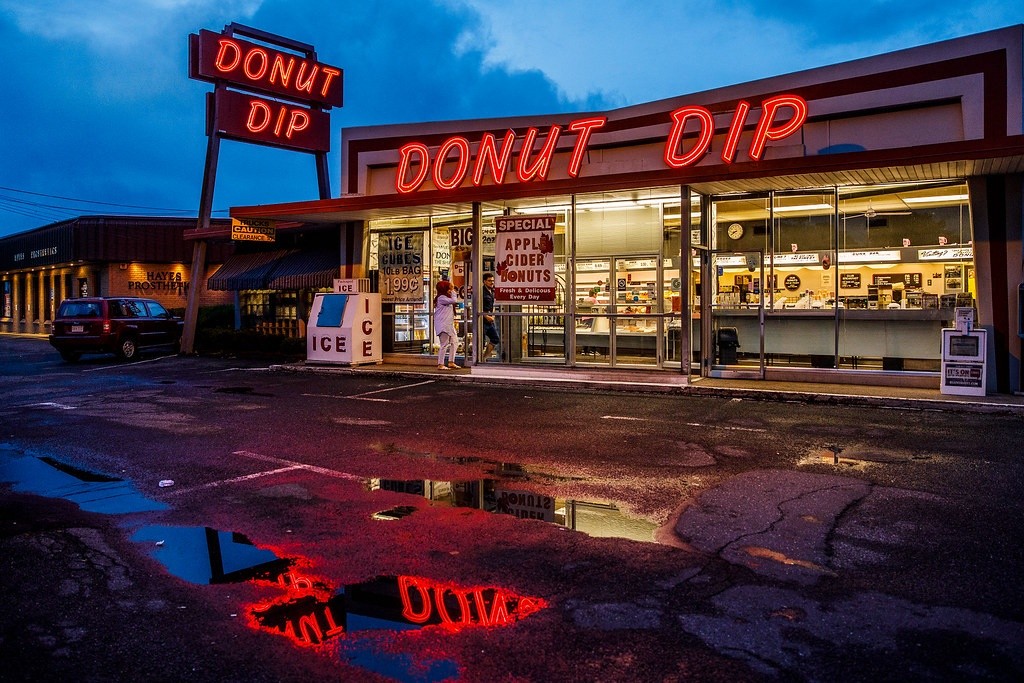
432 282 462 370
482 273 499 362
581 290 602 356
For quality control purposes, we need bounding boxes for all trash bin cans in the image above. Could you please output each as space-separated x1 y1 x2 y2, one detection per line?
717 326 741 366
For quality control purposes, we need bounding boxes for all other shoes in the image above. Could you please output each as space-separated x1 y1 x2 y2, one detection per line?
447 361 461 369
438 364 451 370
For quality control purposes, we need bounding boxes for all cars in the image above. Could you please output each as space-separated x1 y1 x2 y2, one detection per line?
48 295 184 363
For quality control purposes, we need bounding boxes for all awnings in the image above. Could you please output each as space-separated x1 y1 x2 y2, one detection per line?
206 226 342 291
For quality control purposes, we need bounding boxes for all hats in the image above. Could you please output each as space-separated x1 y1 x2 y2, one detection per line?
436 281 451 290
